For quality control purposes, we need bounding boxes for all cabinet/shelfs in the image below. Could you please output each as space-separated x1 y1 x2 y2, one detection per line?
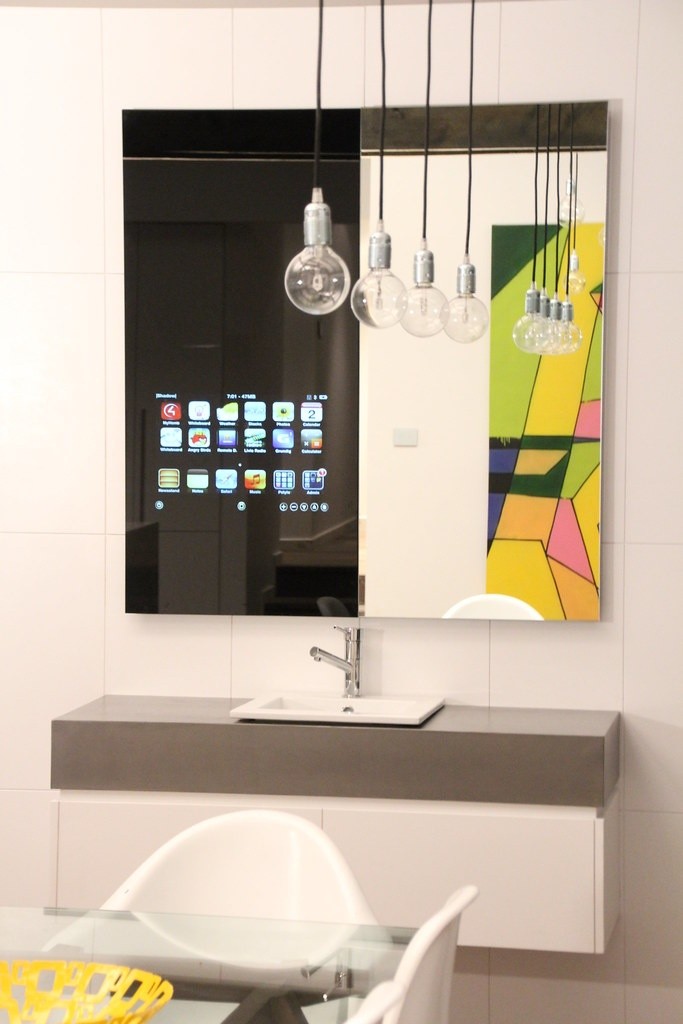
43 794 620 956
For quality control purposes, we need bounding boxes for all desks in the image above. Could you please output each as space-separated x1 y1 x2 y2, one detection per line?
0 903 419 1024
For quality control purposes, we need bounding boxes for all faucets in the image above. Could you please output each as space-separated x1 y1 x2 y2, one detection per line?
310 624 362 698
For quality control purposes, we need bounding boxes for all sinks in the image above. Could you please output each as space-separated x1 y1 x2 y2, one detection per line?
229 693 446 725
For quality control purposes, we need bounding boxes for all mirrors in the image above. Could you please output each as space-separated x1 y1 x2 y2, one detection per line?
360 99 611 623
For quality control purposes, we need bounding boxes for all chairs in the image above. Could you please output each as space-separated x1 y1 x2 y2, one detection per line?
46 812 394 969
349 885 478 1024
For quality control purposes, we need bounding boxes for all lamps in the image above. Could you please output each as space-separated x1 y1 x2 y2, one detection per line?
284 0 490 355
514 102 589 357
